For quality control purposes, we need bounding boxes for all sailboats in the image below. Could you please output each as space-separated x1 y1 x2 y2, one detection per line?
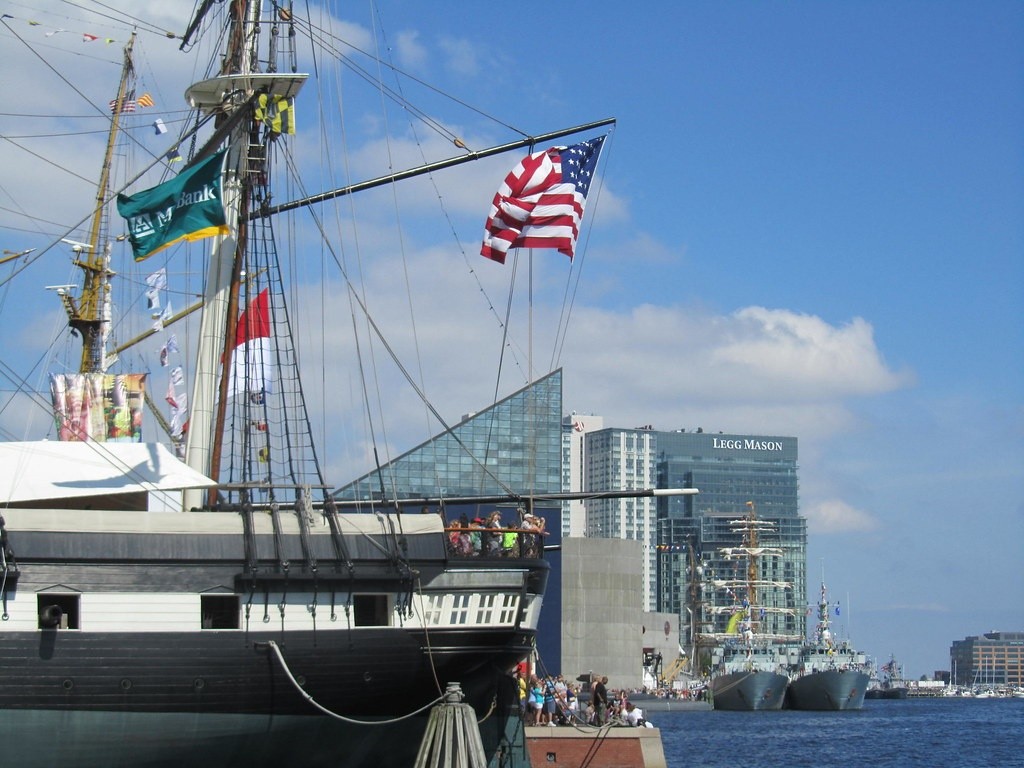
883 653 911 699
1 1 621 768
940 655 1024 699
686 499 811 712
789 560 874 712
862 655 888 699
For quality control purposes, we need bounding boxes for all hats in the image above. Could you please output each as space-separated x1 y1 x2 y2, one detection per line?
474 517 482 523
524 513 533 520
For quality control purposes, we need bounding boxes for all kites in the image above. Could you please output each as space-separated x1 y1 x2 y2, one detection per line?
213 287 271 404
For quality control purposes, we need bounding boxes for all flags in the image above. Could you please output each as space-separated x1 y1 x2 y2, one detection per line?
117 145 231 262
165 150 182 163
137 92 155 106
254 93 295 134
250 392 271 495
479 135 607 264
835 608 840 615
110 89 135 114
154 118 167 135
882 662 889 671
152 301 173 332
146 267 168 291
147 292 160 309
160 334 189 439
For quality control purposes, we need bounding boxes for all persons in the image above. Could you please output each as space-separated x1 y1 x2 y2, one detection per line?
654 685 706 701
518 670 653 727
449 512 545 559
